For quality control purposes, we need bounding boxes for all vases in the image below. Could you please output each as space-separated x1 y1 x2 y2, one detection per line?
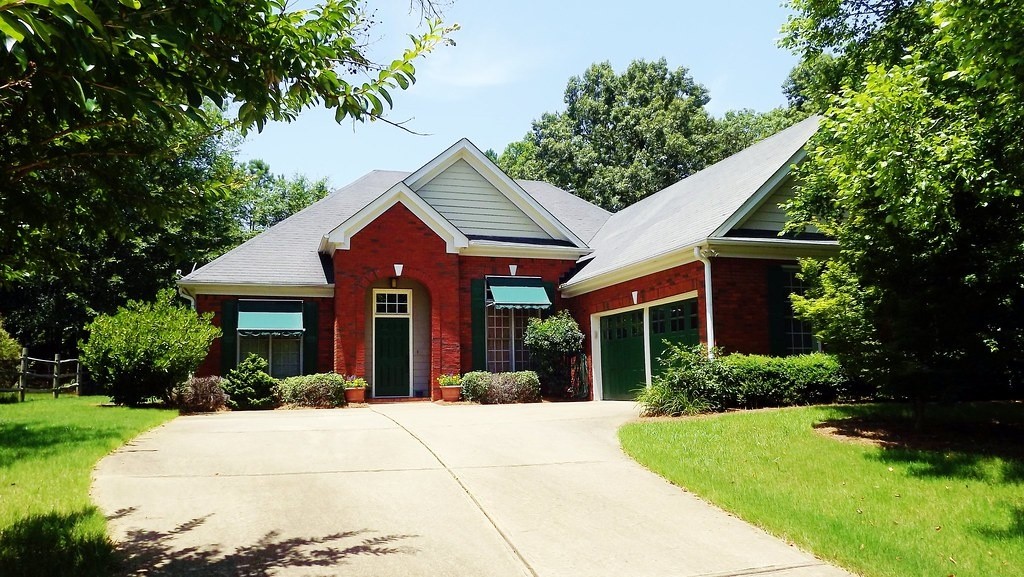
439 386 462 401
345 388 365 403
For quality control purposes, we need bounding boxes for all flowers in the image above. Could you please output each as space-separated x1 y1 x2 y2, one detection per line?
343 374 370 388
437 371 462 386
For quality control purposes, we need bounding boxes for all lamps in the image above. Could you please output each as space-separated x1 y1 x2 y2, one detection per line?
389 277 398 288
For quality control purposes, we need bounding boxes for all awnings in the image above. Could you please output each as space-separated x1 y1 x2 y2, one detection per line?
237 311 305 337
486 276 552 309
